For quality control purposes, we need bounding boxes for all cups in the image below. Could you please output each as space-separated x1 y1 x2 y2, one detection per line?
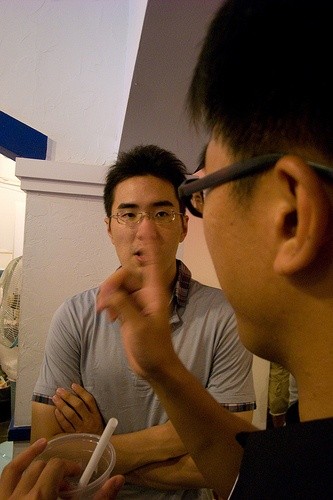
33 433 116 500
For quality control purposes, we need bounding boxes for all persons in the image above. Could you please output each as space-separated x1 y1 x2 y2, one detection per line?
30 144 257 499
1 0 333 499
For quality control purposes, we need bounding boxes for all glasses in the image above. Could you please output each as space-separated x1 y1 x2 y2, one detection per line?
106 208 181 224
178 155 333 219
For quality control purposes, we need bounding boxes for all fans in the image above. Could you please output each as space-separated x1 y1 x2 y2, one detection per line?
0 256 23 349
